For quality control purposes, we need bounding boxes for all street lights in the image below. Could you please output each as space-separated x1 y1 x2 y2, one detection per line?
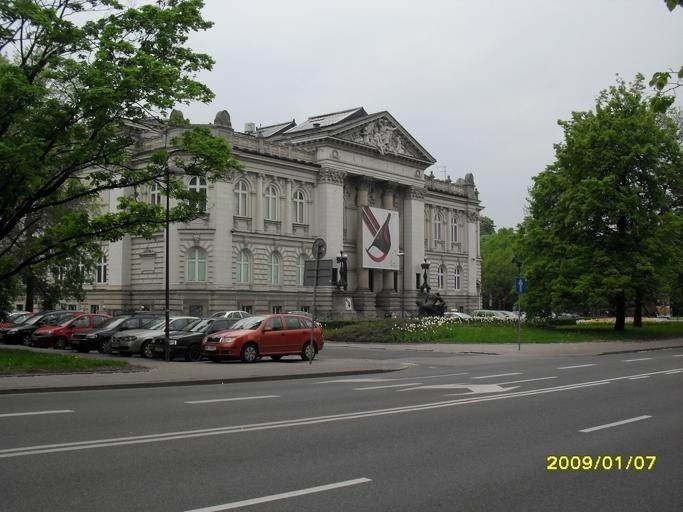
335 248 348 290
511 254 523 350
163 166 186 362
420 257 431 293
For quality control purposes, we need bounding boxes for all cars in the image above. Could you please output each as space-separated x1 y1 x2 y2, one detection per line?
385 311 412 319
445 310 579 322
